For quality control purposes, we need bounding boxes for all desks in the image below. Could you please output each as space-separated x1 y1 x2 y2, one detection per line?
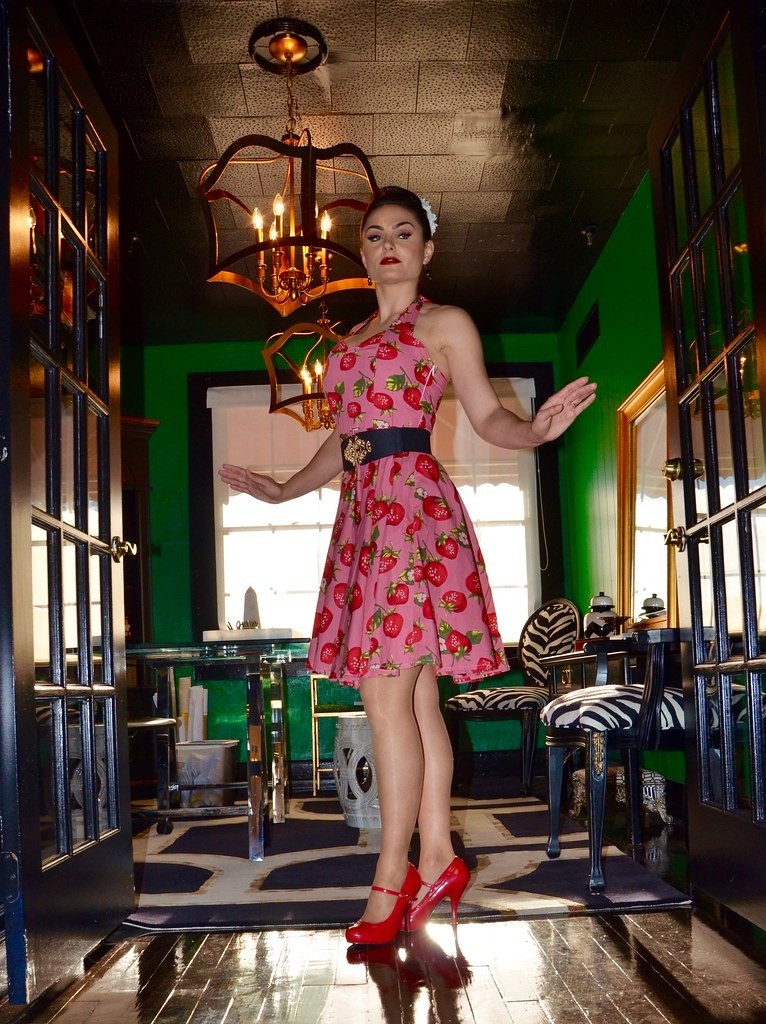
124 638 320 863
572 631 670 841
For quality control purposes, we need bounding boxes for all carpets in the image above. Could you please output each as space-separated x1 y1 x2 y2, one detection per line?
114 794 695 932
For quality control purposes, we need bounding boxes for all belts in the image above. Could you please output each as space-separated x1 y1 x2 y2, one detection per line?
341 428 431 472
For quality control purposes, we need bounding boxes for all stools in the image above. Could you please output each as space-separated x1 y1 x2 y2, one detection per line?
568 765 674 826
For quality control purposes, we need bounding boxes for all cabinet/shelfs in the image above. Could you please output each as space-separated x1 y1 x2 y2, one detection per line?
0 37 143 1007
643 3 766 832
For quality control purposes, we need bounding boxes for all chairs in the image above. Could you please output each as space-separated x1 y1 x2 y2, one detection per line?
440 597 581 786
540 626 680 899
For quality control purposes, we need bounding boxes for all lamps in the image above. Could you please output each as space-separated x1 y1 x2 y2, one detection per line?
196 17 380 432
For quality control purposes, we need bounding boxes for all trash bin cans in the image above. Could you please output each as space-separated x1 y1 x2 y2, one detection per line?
174 739 241 809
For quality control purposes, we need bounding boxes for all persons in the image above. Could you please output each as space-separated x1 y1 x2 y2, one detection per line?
216 187 598 947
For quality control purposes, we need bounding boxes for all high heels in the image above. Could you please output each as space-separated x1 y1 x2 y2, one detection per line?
398 854 472 932
346 860 421 945
